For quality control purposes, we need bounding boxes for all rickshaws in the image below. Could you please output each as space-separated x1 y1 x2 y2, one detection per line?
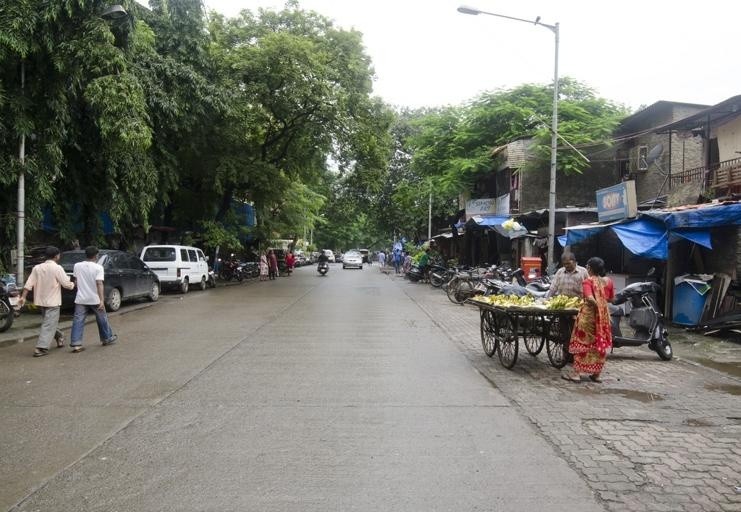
466 293 582 370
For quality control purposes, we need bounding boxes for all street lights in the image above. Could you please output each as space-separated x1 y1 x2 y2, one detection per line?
392 148 433 241
311 211 324 263
15 4 127 291
456 5 559 280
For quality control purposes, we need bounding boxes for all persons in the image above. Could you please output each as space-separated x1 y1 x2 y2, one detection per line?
561 256 617 384
259 249 295 281
545 251 590 364
69 246 118 353
317 251 328 262
369 247 438 285
17 247 77 357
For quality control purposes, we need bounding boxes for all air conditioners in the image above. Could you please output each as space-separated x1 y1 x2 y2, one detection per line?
627 145 649 176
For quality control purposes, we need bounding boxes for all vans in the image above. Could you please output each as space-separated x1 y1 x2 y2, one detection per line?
140 244 209 292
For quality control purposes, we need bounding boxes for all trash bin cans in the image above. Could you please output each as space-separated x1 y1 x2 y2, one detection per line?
672 276 711 326
521 258 542 283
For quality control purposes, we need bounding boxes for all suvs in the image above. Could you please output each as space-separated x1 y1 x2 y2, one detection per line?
266 249 293 273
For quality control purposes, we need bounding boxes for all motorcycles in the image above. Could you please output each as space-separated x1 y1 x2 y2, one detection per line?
1 274 21 332
386 251 553 305
318 256 331 274
203 251 259 288
607 264 675 358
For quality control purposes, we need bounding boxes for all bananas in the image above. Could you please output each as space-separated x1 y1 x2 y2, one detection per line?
487 293 536 306
543 293 584 310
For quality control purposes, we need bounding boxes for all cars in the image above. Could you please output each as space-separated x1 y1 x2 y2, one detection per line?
58 247 162 311
294 248 380 268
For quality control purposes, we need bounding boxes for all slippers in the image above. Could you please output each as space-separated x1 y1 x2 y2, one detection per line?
589 376 604 384
561 373 582 384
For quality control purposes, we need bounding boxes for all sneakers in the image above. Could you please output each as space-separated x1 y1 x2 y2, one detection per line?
56 331 66 348
101 335 119 346
69 344 87 355
32 347 49 357
